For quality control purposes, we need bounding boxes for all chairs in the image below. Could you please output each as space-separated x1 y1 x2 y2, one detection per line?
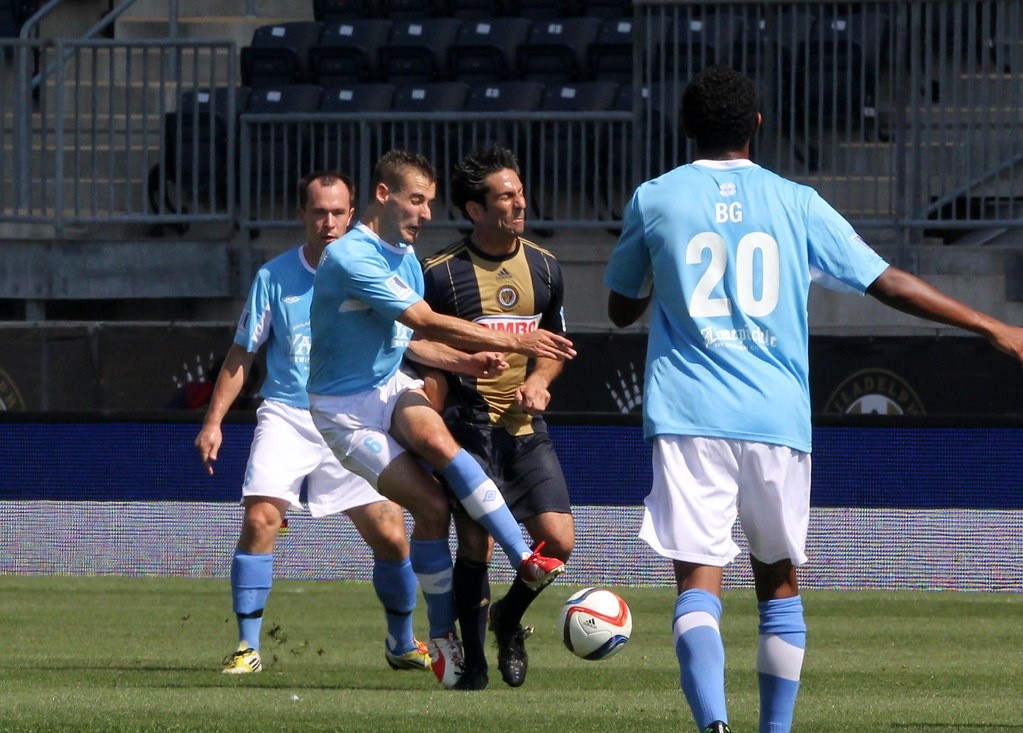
146 3 933 244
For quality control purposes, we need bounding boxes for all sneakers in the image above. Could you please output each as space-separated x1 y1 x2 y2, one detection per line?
427 632 466 691
453 653 489 692
384 637 433 672
490 598 530 688
219 640 263 675
518 550 567 592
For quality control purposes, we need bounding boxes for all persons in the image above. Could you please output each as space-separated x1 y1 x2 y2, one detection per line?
606 64 1023 733
306 151 565 688
408 143 574 689
195 173 429 675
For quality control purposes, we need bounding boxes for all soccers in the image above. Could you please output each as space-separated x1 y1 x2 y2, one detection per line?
557 586 634 662
